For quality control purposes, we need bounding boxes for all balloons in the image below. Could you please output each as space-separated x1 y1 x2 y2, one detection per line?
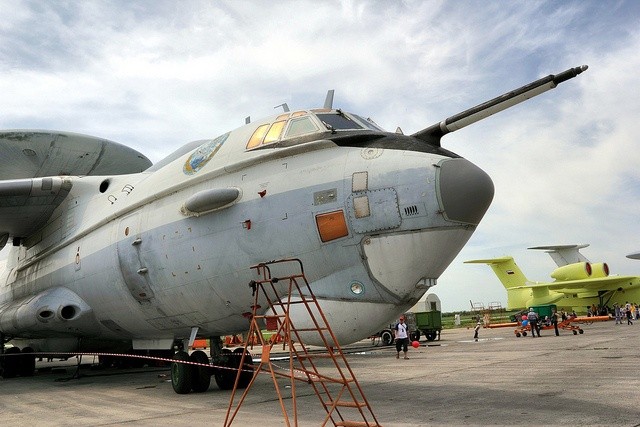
522 315 527 321
522 320 527 327
411 341 420 348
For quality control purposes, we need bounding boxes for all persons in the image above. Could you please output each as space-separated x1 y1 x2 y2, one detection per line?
572 310 577 318
592 303 597 316
625 301 633 325
561 308 565 322
551 308 560 336
634 303 638 311
630 303 636 320
543 318 550 327
394 315 410 359
612 302 623 324
587 306 591 317
527 307 542 337
619 304 623 315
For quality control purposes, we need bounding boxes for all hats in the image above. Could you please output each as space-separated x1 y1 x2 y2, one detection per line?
400 316 403 320
530 308 534 312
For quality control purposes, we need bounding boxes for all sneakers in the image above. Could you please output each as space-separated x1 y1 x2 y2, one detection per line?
404 356 409 359
396 354 399 359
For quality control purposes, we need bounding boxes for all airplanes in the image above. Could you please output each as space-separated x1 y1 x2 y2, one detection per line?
463 255 640 319
0 63 589 394
527 243 640 284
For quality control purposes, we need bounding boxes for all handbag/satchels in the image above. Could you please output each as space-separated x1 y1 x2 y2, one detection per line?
550 315 556 322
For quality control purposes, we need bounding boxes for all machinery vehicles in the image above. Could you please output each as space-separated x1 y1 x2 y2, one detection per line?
366 293 441 346
